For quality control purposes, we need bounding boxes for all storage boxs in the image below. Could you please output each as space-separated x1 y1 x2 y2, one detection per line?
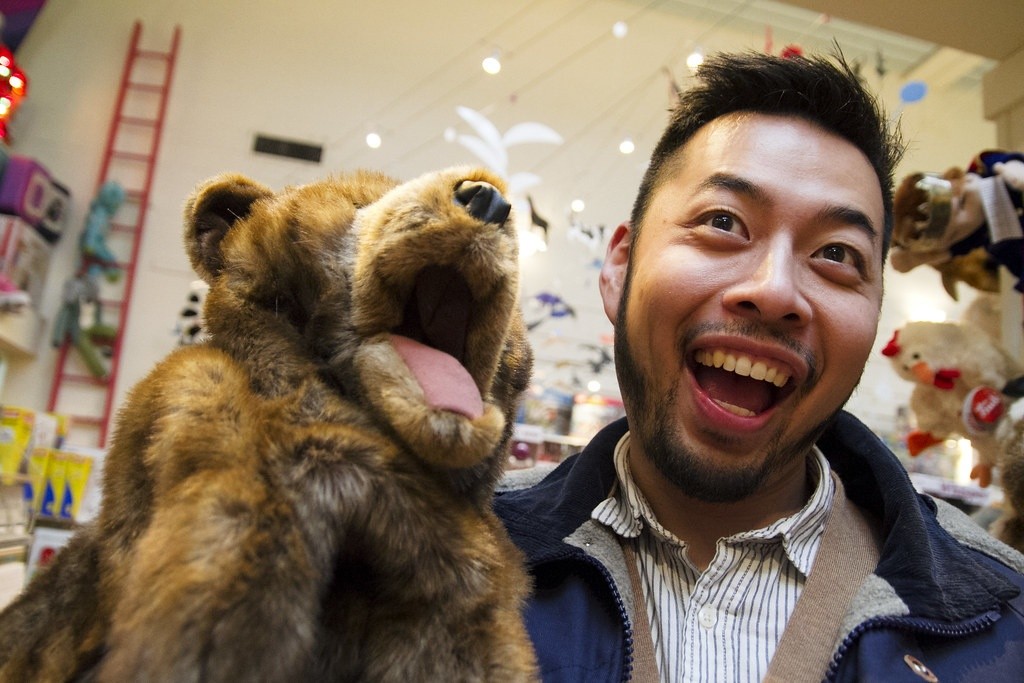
0 155 98 546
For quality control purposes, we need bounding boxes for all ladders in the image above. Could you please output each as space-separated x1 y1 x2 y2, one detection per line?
43 21 181 448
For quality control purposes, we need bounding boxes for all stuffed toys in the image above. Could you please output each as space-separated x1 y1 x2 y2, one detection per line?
883 295 1023 488
0 163 542 682
889 146 1023 298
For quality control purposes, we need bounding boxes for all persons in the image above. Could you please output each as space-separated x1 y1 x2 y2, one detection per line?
492 39 1024 683
